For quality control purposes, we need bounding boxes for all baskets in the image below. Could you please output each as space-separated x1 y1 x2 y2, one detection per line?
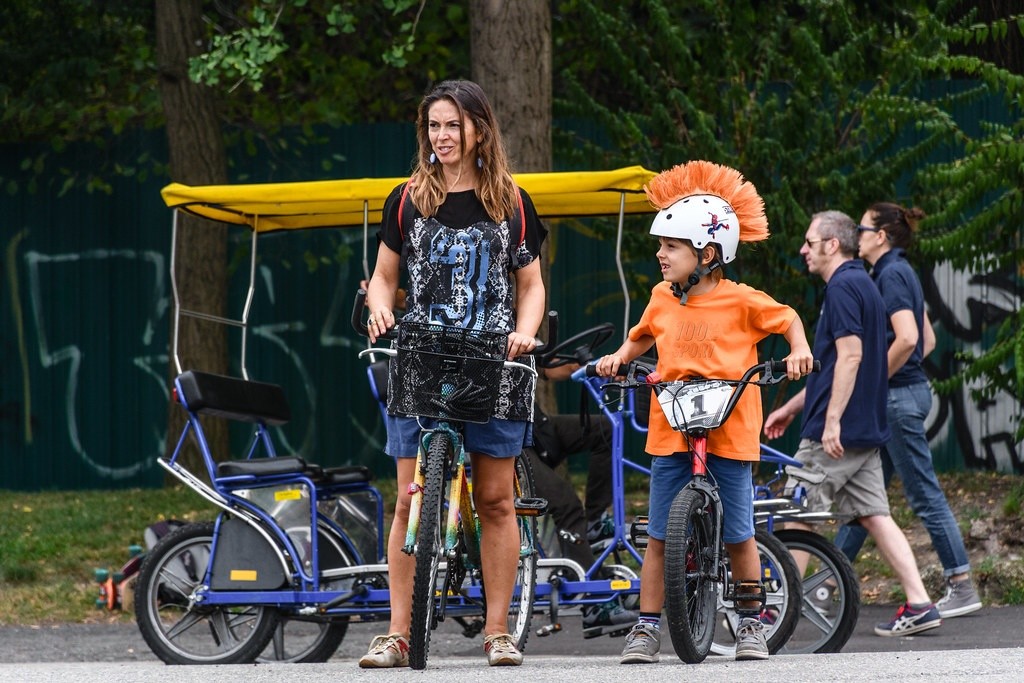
389 320 509 424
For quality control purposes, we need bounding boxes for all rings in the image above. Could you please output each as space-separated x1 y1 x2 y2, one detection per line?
369 319 374 325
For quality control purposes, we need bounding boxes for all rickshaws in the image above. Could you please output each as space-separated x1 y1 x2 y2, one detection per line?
124 162 862 667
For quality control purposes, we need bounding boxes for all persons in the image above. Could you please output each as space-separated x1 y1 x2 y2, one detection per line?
358 280 640 639
799 202 984 619
595 162 815 667
720 210 944 636
359 78 549 667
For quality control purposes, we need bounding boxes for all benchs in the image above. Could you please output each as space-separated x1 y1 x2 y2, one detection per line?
175 370 384 592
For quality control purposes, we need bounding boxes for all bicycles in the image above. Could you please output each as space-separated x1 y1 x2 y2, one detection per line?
586 357 821 665
360 320 552 668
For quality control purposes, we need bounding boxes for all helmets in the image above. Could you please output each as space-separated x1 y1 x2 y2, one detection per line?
643 161 769 266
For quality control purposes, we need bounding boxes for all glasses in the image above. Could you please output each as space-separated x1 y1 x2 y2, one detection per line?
805 238 833 247
857 224 893 240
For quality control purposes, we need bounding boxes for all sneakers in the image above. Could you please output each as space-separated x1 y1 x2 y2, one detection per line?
356 634 409 666
760 610 778 626
621 623 661 664
734 617 769 659
798 580 836 615
581 604 640 638
587 510 632 553
932 576 981 618
873 600 940 637
482 633 522 666
